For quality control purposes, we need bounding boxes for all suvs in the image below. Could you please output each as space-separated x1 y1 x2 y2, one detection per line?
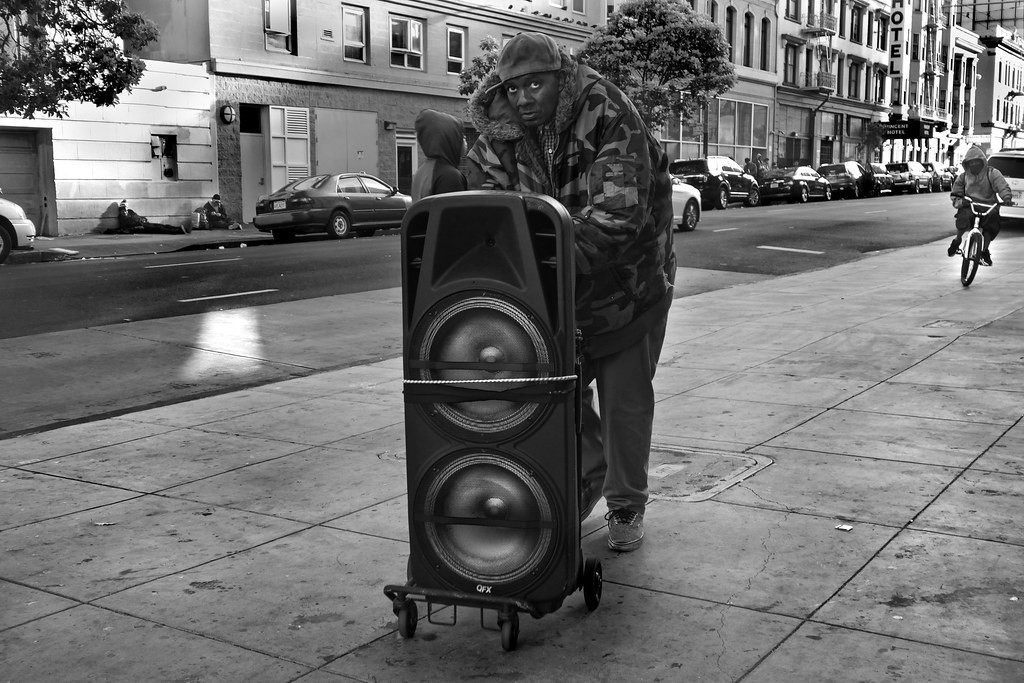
817 161 875 199
870 161 961 194
668 156 760 210
985 150 1024 219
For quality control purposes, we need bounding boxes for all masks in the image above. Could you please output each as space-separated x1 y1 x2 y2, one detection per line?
970 162 983 175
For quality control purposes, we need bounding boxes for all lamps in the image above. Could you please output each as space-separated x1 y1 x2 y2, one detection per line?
384 121 397 130
220 105 236 124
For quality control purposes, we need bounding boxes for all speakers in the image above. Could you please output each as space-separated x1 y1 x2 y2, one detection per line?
401 190 582 605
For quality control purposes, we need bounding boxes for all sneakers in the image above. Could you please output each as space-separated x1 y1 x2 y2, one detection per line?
604 507 643 549
578 477 607 519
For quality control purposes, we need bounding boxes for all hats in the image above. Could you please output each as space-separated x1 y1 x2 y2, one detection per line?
212 194 220 200
484 32 561 94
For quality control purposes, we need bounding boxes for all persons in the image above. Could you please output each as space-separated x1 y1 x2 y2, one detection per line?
948 144 1013 266
205 194 242 230
744 158 756 179
457 31 678 554
756 153 769 177
410 108 467 202
119 207 192 234
771 162 779 169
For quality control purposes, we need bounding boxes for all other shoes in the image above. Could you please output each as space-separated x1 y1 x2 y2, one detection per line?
179 220 193 235
229 223 242 230
948 238 962 257
980 251 992 266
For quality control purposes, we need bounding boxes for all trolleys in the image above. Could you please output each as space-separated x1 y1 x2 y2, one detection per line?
382 189 603 653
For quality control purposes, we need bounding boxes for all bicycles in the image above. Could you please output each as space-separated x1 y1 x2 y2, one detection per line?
948 193 1016 286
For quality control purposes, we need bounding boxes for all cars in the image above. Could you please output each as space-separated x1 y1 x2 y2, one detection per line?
253 171 412 243
670 175 702 231
0 198 36 264
760 166 831 203
